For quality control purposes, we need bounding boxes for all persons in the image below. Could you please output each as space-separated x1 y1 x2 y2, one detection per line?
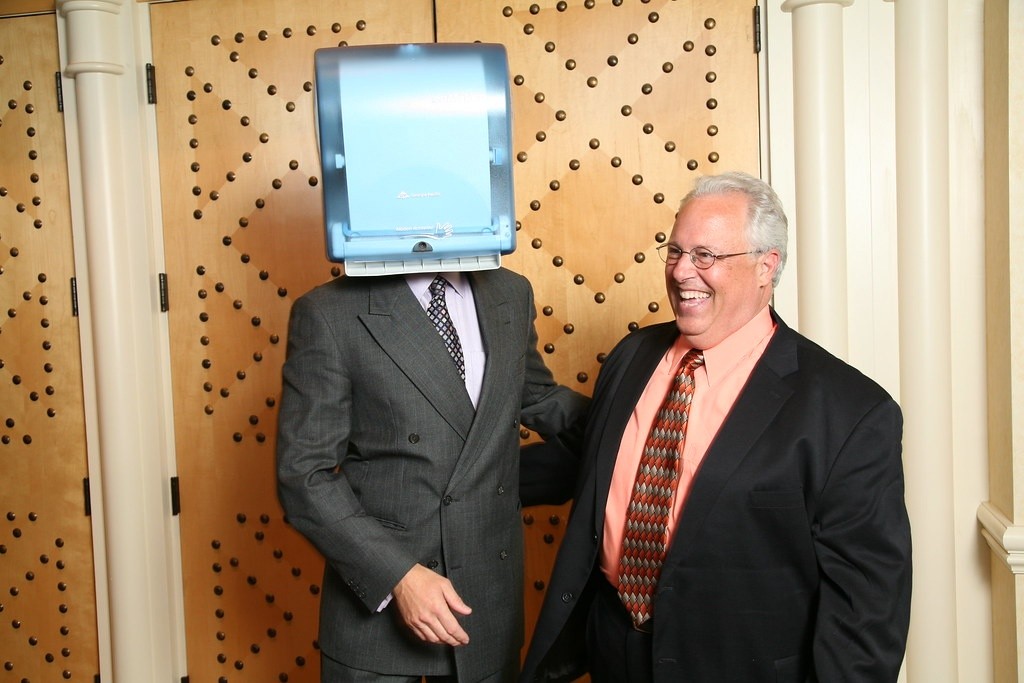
519 173 912 683
277 267 595 683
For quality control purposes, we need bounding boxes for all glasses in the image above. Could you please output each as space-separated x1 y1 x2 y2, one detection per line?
656 243 761 270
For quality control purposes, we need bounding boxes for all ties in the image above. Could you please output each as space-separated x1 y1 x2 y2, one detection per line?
617 349 706 630
426 275 466 385
